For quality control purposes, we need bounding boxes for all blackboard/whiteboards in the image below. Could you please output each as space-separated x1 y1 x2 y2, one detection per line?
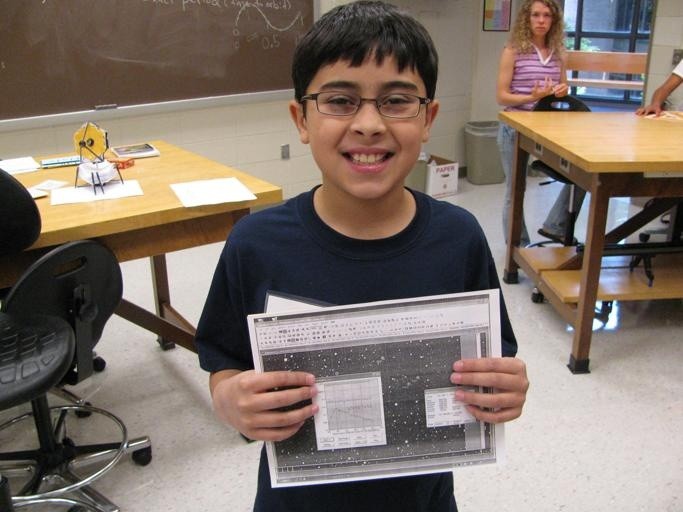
0 0 322 132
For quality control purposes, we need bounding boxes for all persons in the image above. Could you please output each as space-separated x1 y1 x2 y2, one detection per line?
634 59 683 118
194 1 529 510
496 1 586 250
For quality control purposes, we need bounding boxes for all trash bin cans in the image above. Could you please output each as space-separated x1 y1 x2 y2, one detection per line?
464 121 505 185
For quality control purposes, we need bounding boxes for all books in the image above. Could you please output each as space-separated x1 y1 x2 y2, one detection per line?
109 143 161 160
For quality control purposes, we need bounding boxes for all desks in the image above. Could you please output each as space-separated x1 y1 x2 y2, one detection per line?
0 139 285 442
496 110 683 374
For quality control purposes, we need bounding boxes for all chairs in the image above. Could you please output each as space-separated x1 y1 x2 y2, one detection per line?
0 168 152 512
639 204 677 242
522 91 613 314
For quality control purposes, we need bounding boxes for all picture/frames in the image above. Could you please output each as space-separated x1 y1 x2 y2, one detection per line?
483 0 512 32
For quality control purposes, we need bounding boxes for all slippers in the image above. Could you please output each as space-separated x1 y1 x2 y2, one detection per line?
537 227 578 246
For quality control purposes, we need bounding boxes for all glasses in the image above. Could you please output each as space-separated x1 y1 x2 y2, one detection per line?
300 90 434 117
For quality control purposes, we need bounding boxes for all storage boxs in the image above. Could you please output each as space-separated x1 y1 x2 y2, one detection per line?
405 151 458 200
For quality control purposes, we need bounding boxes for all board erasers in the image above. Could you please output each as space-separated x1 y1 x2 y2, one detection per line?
95 103 118 110
